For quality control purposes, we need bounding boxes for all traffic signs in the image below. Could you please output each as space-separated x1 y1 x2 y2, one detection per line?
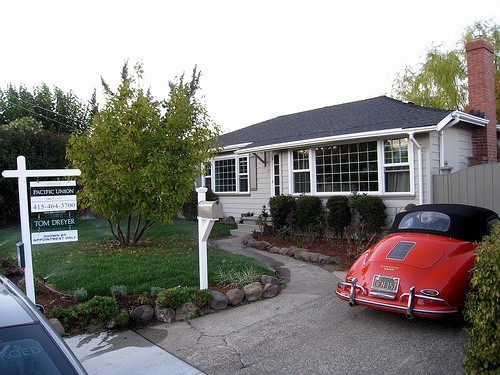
30 180 79 245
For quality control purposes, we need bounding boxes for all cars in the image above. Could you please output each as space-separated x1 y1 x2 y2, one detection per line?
333 203 499 332
1 276 89 374
